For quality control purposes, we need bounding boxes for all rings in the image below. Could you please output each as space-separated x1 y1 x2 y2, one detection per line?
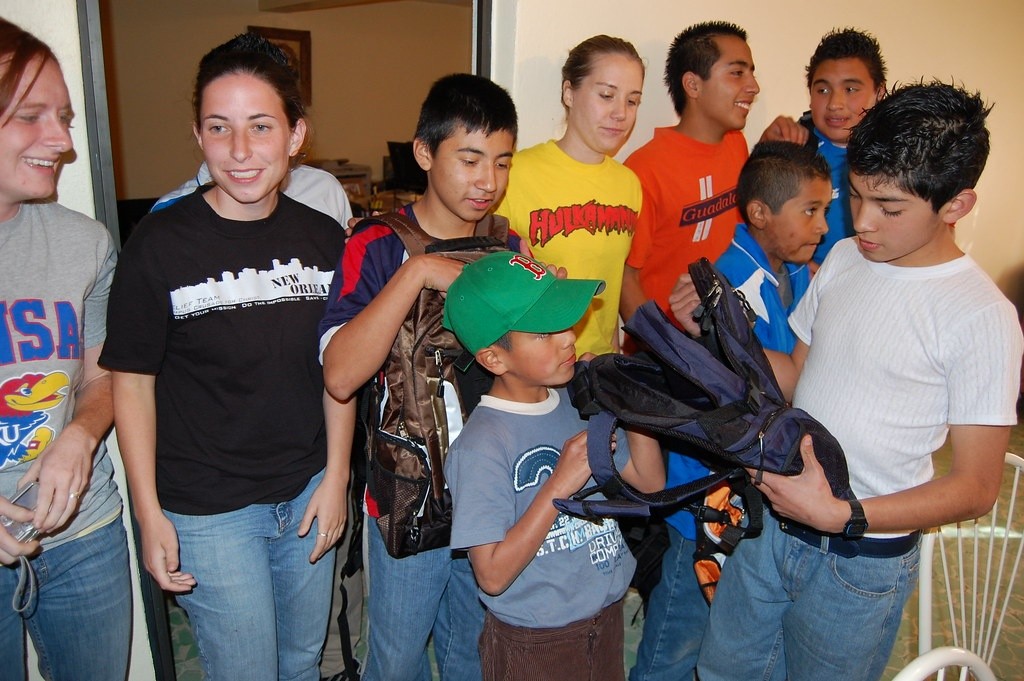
317 532 329 537
68 491 80 499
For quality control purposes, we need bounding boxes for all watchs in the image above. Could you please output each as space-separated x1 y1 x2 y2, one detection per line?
842 498 868 538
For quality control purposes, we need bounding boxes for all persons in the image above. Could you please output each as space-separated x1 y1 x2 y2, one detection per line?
151 153 354 235
500 35 644 373
319 71 567 681
0 17 133 681
667 73 1024 681
96 33 356 681
442 239 640 681
797 27 889 287
629 140 834 681
623 22 808 616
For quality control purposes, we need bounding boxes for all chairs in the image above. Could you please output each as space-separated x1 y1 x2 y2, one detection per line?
891 452 1024 681
382 140 428 211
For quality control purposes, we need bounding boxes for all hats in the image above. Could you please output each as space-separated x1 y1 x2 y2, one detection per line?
442 250 605 371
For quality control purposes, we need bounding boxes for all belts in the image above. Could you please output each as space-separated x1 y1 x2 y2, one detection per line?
775 516 918 558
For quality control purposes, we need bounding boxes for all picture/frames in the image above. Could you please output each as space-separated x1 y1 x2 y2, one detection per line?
246 24 313 107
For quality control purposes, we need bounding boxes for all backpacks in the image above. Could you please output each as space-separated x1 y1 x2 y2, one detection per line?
568 256 853 515
354 212 511 559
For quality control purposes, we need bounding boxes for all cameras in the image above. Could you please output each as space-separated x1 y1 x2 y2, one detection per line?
0 480 43 543
